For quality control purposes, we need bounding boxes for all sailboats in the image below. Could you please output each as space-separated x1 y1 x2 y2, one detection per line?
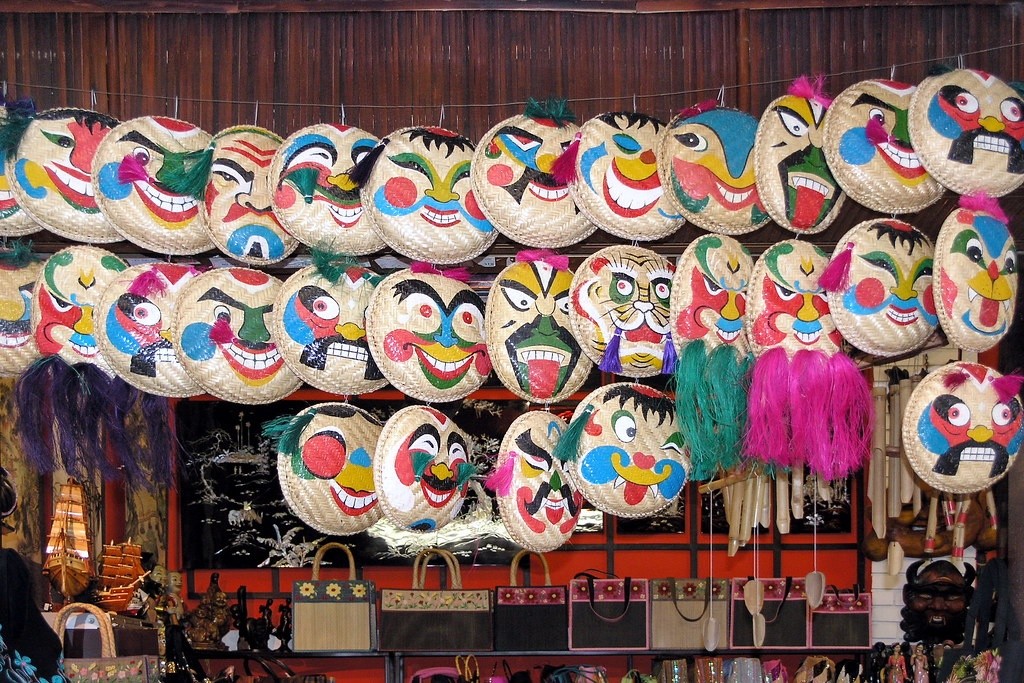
42 477 95 613
89 537 150 611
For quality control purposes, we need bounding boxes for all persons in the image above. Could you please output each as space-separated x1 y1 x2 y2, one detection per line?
885 643 909 683
910 643 930 683
128 565 176 625
166 570 188 625
259 597 293 633
936 639 955 682
0 466 71 683
183 591 232 645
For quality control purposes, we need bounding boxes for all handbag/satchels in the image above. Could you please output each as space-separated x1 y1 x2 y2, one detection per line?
494 549 569 651
52 603 160 683
567 572 651 650
166 624 328 683
378 548 492 652
646 577 729 651
400 641 914 683
729 575 811 649
936 558 1024 683
291 543 377 656
808 583 874 650
